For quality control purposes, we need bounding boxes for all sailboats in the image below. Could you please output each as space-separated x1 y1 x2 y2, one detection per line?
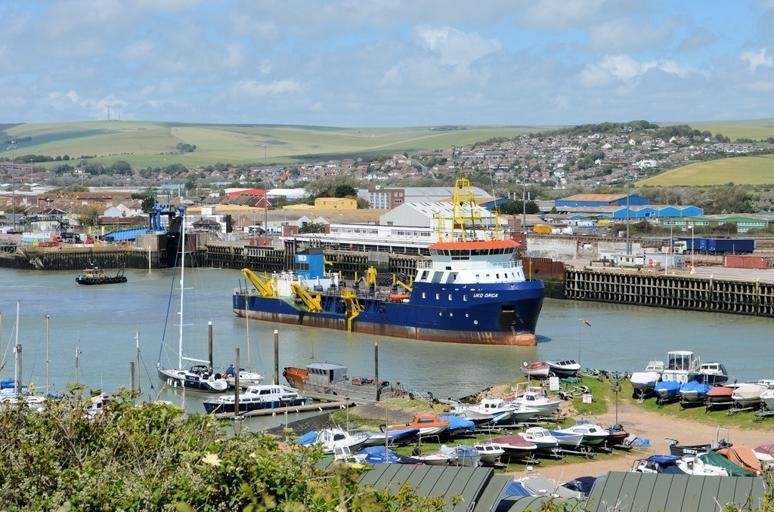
155 204 233 393
281 341 414 408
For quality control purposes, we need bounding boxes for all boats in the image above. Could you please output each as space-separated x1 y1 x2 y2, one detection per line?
0 297 52 417
202 377 316 418
546 359 582 378
629 347 774 424
519 360 556 382
74 261 129 286
216 291 267 391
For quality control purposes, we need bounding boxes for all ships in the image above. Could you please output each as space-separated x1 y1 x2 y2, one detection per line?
228 173 547 347
